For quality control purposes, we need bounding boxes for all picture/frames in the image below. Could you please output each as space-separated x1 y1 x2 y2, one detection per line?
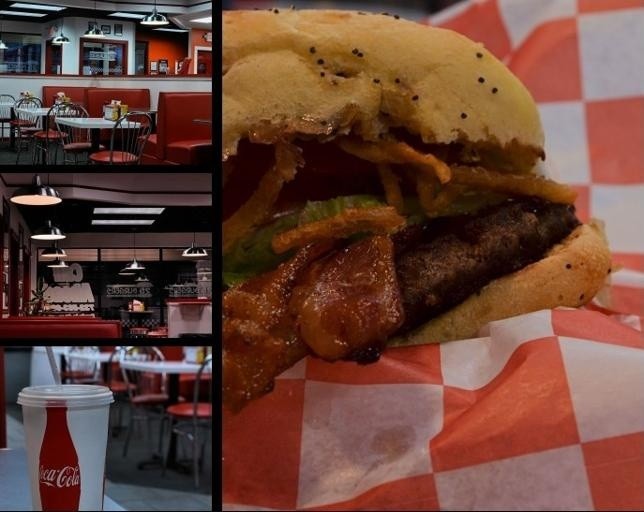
114 23 123 36
101 25 111 34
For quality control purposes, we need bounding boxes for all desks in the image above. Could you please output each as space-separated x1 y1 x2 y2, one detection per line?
0 448 125 511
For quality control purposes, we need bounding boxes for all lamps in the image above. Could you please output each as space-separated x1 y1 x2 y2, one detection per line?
118 231 208 275
53 23 72 43
83 16 103 39
140 0 168 24
11 177 69 268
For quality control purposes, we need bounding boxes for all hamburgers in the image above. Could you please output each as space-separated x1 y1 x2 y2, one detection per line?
221 9 613 414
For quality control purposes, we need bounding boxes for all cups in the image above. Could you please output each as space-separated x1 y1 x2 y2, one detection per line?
17 383 115 512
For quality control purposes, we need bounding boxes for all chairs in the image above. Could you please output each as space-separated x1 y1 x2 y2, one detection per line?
31 344 212 486
0 87 212 165
